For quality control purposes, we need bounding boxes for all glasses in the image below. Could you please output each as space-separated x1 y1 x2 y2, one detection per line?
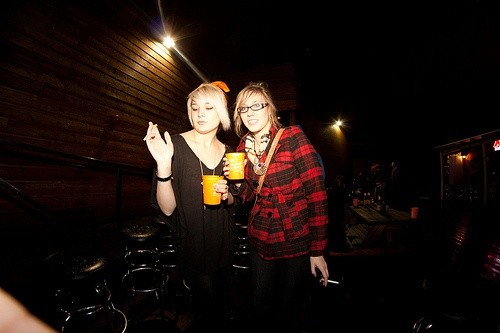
236 102 269 113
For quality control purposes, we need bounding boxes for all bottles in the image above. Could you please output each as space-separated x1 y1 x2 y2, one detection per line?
363 193 366 205
367 192 370 205
377 196 381 211
353 193 359 208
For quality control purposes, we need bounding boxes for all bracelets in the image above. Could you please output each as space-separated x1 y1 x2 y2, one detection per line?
155 170 173 182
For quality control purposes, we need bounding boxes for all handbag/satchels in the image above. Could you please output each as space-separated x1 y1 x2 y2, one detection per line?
231 223 249 268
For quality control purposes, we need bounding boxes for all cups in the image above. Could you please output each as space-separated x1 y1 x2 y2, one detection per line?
411 208 418 218
202 175 224 205
225 153 245 179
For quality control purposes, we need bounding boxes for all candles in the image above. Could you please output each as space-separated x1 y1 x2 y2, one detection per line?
353 198 359 207
410 207 419 219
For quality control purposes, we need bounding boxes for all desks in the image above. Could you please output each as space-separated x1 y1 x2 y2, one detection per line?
350 204 416 249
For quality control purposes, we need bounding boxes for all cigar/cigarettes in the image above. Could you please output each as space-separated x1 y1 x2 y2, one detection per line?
143 124 158 141
321 279 340 284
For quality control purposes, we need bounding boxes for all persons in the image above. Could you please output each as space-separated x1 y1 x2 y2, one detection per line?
224 83 329 333
376 177 419 219
146 84 237 333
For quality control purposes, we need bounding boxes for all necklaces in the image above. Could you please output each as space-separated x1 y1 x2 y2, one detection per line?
249 132 270 175
